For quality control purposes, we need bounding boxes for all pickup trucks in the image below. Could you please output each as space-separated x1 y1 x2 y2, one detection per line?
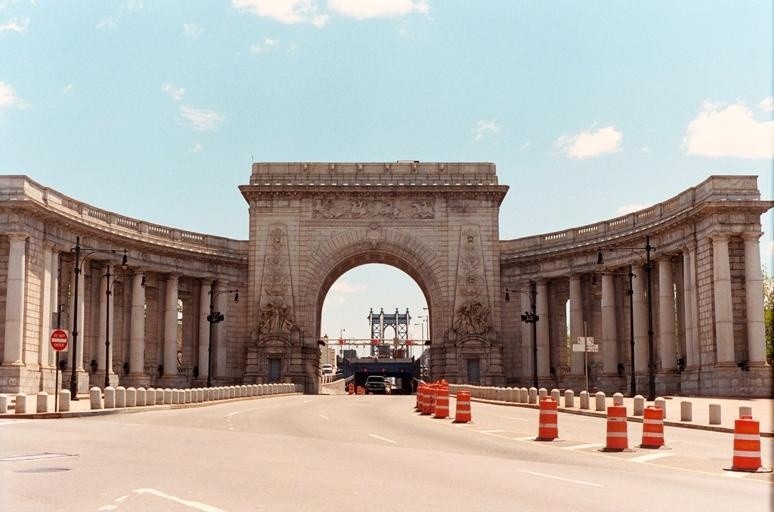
364 375 385 394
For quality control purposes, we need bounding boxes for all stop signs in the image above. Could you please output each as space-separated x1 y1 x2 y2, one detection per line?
50 329 68 352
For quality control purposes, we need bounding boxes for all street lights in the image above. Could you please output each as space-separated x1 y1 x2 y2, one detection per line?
591 263 638 399
594 233 659 404
68 234 129 402
339 327 345 371
206 282 240 389
101 263 148 400
413 306 429 375
503 277 540 397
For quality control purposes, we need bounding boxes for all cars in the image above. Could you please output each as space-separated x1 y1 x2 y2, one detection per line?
321 363 333 374
335 368 343 374
